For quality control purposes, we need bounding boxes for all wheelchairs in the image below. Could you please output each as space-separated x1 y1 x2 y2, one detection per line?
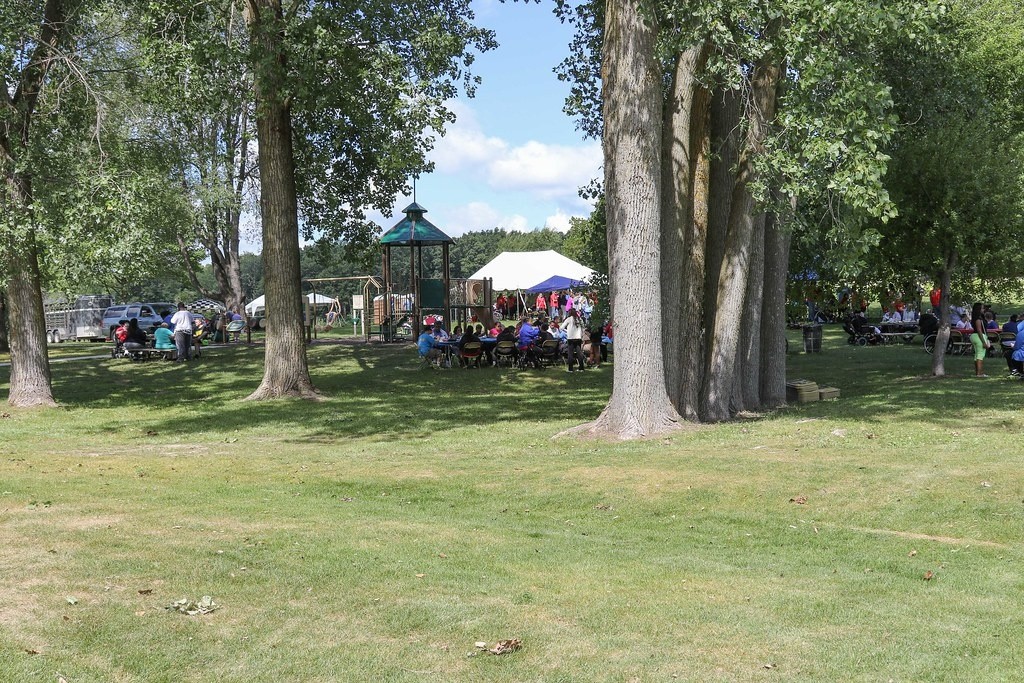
919 314 963 355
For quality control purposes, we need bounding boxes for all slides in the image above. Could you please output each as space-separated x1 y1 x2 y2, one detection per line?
468 283 506 330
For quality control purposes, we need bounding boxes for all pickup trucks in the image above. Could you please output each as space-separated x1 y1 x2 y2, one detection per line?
101 303 204 340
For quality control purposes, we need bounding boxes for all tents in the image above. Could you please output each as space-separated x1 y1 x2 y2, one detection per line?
245 294 266 317
525 275 591 294
452 250 610 321
185 299 226 314
306 293 342 329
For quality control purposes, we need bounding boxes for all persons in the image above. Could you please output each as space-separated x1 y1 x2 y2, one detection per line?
949 301 973 353
403 290 613 373
805 279 866 324
878 284 922 313
116 302 243 362
969 303 989 377
930 284 941 317
984 305 1024 377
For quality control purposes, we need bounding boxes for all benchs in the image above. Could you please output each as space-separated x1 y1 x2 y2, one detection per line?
882 332 921 346
127 348 177 362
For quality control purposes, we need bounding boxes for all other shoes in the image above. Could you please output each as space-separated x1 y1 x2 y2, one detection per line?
977 374 989 377
463 365 468 369
566 370 573 373
577 369 584 372
1007 369 1024 378
473 365 477 368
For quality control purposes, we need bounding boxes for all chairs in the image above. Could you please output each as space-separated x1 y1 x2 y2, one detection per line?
413 339 585 370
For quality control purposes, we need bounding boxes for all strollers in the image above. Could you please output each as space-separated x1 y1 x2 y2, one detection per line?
844 315 881 346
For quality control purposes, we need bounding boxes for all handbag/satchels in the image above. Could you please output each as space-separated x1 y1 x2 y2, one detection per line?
987 340 990 348
585 307 591 312
536 308 539 311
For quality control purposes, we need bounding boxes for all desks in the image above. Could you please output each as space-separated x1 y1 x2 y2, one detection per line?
881 321 919 332
438 337 497 370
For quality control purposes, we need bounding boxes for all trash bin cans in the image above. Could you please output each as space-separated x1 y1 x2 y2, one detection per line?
800 322 823 353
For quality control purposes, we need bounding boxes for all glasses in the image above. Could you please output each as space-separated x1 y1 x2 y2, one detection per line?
498 326 502 327
